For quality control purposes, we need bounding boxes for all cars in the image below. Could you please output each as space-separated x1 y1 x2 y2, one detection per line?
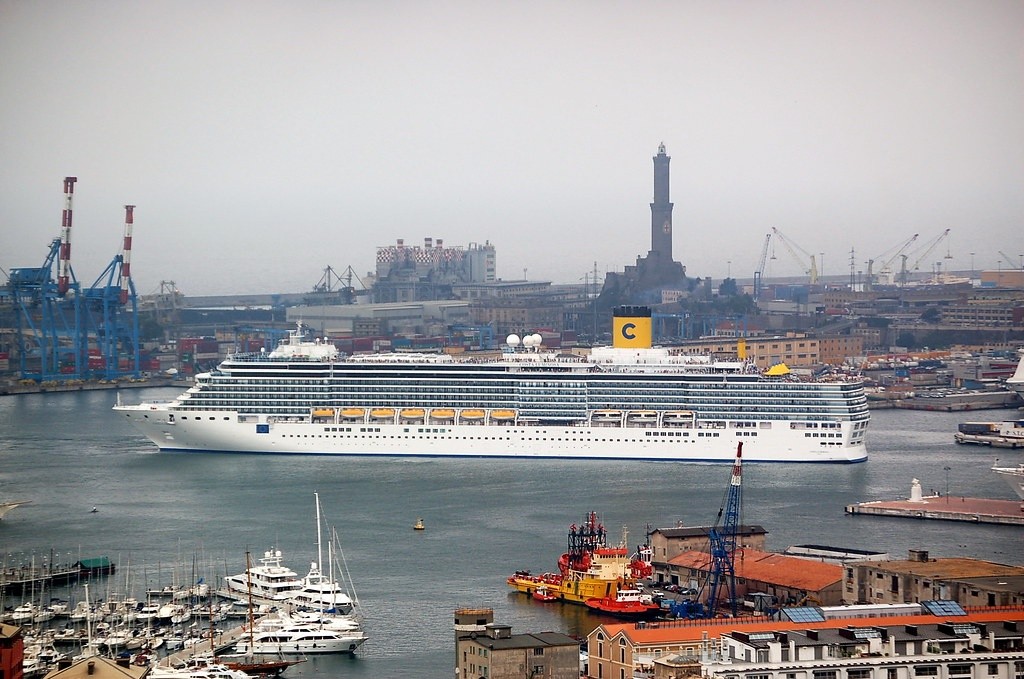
648 581 697 595
651 590 664 598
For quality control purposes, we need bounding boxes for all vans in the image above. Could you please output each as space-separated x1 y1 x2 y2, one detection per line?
635 582 644 591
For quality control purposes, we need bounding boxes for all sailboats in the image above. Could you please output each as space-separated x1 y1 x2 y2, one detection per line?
279 493 359 614
0 543 310 679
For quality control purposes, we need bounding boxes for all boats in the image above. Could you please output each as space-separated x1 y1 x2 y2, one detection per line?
400 409 454 420
224 544 304 601
233 610 372 655
313 408 333 417
506 508 660 617
371 407 394 418
461 408 515 420
341 409 364 418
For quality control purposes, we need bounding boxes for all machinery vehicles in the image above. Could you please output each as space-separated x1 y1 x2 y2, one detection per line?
777 594 823 608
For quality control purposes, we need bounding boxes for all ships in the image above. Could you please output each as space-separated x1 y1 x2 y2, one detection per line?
111 313 871 466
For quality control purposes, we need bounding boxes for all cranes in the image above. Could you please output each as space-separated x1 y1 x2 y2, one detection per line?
865 227 950 284
771 226 819 284
756 234 772 295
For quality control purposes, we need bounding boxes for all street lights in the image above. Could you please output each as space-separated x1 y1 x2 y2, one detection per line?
945 466 952 503
